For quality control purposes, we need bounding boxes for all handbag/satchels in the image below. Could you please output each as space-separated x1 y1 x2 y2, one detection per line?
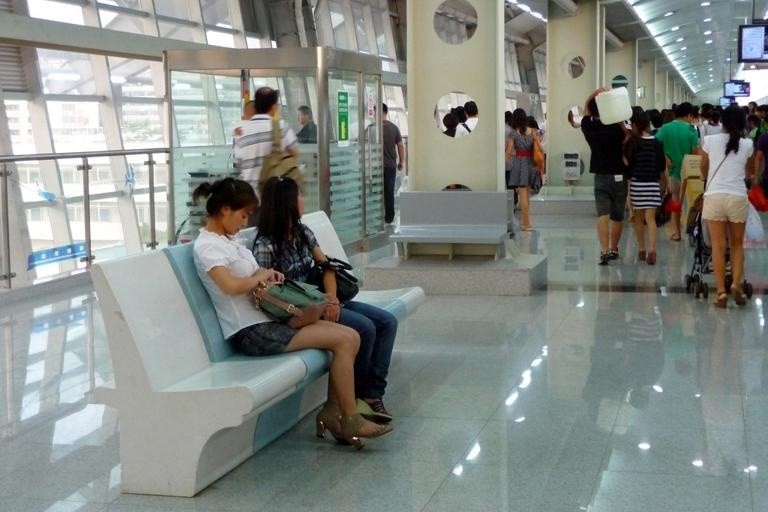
747 185 768 212
304 257 361 301
533 129 545 164
251 276 329 329
655 193 672 226
693 193 705 210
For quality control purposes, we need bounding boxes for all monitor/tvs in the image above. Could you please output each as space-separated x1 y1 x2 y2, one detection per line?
719 81 750 106
738 24 768 63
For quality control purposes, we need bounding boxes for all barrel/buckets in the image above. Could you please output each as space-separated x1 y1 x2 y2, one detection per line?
594 87 633 125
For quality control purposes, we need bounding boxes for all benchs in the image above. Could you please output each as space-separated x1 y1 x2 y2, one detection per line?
85 210 427 498
504 187 521 252
385 189 510 262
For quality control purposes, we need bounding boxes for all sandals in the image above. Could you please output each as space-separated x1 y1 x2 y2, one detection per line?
597 247 621 264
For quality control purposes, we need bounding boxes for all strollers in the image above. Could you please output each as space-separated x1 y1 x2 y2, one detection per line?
680 191 756 300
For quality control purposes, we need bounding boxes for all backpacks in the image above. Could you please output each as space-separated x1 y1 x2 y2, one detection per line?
256 148 306 197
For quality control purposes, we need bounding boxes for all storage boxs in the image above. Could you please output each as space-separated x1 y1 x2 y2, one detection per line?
677 152 704 201
679 177 708 236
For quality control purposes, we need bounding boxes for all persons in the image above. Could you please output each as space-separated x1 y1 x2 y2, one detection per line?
253 176 399 421
232 99 256 137
231 86 301 225
295 104 317 143
192 177 394 449
366 103 405 223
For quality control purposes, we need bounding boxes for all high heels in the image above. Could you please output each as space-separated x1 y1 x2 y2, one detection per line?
341 414 391 450
731 283 749 305
649 250 657 265
640 249 646 261
357 396 392 422
713 291 729 310
314 401 348 448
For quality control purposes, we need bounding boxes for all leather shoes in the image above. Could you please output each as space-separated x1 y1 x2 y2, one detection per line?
509 223 534 237
